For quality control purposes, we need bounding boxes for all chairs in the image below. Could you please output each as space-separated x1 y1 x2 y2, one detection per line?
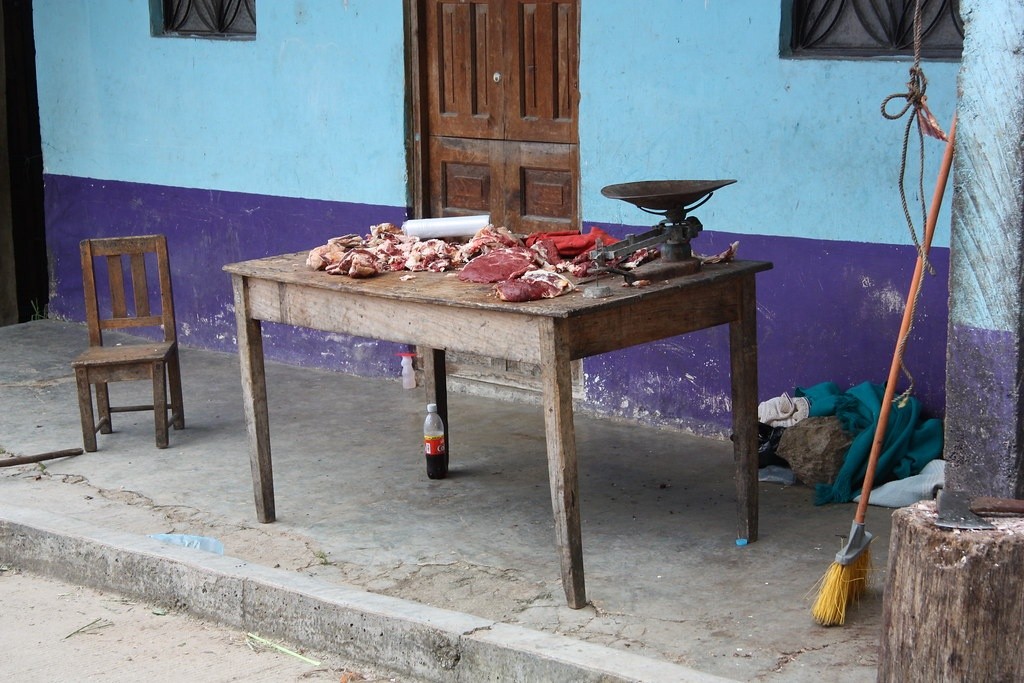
69 235 185 451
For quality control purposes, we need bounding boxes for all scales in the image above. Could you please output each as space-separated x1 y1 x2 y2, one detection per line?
582 179 738 300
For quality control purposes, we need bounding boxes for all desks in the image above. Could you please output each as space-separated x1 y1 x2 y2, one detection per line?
220 224 774 611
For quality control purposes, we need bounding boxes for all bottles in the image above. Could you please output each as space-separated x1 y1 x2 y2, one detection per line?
401 356 417 390
424 403 448 479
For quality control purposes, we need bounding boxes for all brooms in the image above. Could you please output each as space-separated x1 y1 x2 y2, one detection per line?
808 108 961 628
